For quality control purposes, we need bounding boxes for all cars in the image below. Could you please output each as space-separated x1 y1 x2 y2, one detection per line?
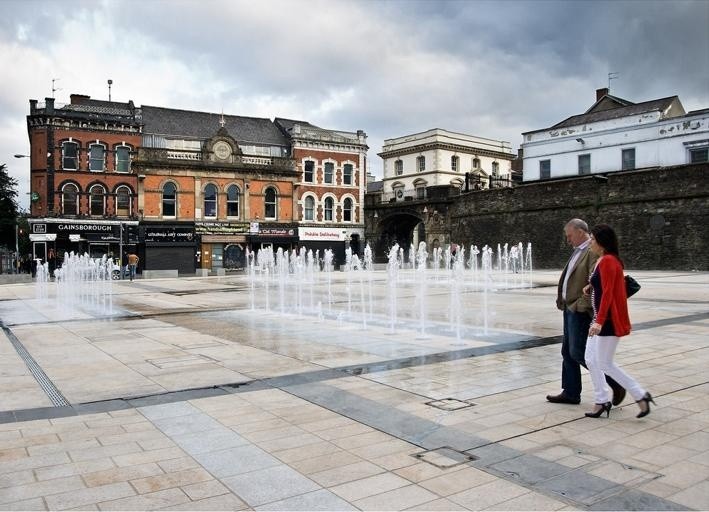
111 265 130 280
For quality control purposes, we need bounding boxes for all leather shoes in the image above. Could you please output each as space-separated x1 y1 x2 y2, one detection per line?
547 390 580 404
613 386 625 405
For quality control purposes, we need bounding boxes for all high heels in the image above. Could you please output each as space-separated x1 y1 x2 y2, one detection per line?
636 392 656 418
585 402 612 418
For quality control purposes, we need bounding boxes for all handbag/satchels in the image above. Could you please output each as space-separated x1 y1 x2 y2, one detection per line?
625 275 641 298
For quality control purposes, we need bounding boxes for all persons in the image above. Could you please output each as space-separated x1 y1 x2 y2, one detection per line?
582 223 657 418
13 258 31 274
546 217 626 406
126 250 139 282
121 251 127 278
